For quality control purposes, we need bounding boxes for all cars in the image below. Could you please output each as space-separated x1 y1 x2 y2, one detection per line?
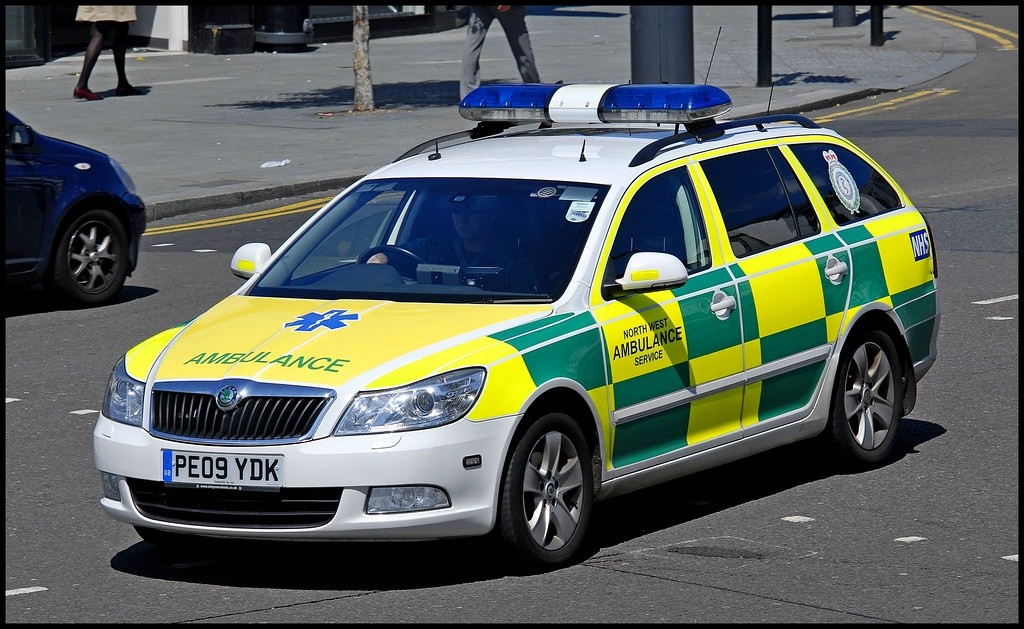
5 109 148 310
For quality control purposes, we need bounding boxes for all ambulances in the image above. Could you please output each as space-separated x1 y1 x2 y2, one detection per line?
90 75 941 574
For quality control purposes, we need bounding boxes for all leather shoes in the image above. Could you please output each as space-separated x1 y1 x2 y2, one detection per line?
73 87 105 100
114 84 152 96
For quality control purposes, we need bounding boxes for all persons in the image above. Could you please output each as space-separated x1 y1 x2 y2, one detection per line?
367 190 525 292
460 4 562 103
73 5 142 100
